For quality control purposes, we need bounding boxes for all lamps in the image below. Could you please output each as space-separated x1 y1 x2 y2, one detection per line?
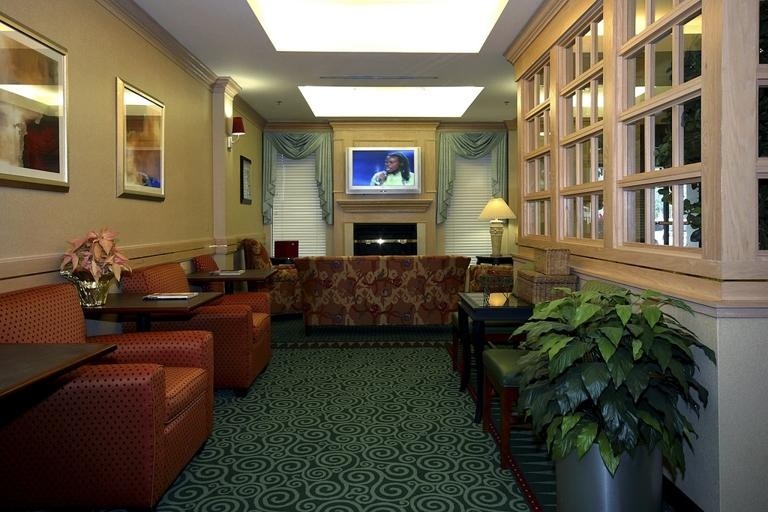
477 199 517 257
227 117 245 150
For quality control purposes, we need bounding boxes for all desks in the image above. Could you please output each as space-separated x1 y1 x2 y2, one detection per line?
476 254 514 267
83 292 223 332
458 291 532 425
1 342 117 399
186 269 278 293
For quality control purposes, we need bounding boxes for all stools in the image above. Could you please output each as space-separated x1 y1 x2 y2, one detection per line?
484 347 531 469
453 313 522 373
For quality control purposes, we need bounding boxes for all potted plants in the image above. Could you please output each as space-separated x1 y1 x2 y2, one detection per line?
511 281 716 512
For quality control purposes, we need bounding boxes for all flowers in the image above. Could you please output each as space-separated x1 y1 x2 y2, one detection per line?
60 226 132 283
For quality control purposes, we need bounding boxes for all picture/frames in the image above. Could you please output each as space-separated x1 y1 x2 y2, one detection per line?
116 77 165 201
0 12 70 192
240 156 252 204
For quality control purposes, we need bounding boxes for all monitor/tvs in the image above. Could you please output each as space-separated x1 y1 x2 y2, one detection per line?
345 146 422 194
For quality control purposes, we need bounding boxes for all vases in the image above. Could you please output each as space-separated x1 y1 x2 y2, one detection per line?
63 273 113 306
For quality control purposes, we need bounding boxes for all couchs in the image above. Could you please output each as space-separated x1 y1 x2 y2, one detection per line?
295 254 471 335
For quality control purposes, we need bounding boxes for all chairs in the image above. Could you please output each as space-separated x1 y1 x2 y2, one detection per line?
1 283 214 510
243 240 303 319
192 256 224 291
117 261 271 397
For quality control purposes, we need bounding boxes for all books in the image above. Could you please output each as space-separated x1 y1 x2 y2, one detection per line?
215 269 245 275
142 292 199 300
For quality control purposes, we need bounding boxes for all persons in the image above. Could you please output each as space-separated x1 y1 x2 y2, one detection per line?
369 152 414 187
